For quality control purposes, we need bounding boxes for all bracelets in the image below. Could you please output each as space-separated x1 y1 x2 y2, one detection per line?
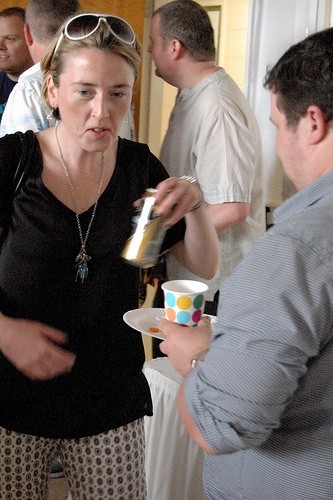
190 348 210 368
178 175 204 213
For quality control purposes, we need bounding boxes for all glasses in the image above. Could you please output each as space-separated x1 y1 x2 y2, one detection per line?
51 13 136 62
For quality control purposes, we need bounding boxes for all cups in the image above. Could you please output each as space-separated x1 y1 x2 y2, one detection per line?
161 280 210 327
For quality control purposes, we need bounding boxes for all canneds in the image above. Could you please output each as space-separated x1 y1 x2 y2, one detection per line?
120 188 171 268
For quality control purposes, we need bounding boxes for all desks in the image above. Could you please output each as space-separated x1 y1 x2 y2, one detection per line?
142 356 207 500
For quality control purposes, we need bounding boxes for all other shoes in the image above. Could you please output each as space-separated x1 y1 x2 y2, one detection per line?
49 458 62 479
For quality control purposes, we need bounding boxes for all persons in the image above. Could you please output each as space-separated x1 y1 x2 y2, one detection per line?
0 6 35 125
158 26 333 500
54 121 106 283
147 0 266 360
1 13 221 500
0 0 136 479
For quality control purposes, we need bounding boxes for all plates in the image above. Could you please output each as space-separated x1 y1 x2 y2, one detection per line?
123 308 218 340
